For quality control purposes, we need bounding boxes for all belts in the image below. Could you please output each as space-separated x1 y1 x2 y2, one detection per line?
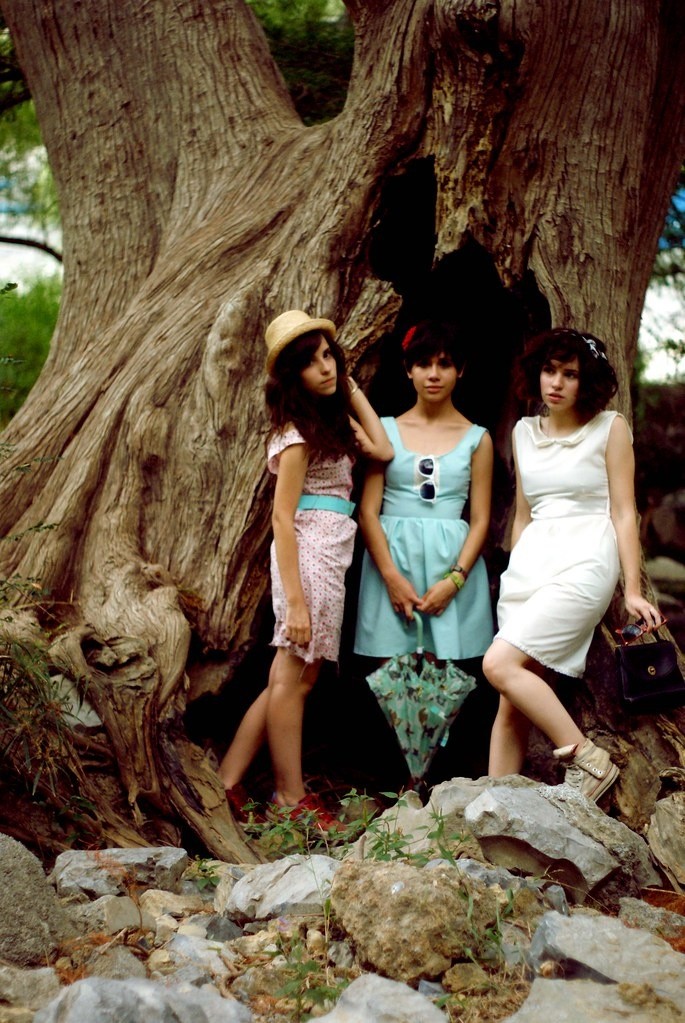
296 493 356 517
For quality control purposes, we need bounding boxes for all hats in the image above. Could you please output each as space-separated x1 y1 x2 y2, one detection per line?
265 310 336 371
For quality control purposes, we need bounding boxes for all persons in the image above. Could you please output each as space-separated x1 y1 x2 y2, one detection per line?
481 327 663 806
353 319 494 793
219 310 395 832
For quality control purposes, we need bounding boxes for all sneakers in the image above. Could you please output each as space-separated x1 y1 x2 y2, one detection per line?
266 791 354 843
553 738 620 804
225 784 271 833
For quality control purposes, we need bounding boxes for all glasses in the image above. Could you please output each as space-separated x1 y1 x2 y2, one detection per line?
615 616 667 647
415 454 439 505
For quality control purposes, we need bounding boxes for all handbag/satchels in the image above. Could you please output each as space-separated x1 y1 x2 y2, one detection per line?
616 620 685 715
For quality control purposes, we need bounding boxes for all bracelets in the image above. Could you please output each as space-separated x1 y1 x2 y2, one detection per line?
449 562 467 580
443 572 463 590
350 386 359 396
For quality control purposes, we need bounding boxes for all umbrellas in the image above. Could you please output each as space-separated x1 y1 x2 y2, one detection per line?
365 612 476 792
